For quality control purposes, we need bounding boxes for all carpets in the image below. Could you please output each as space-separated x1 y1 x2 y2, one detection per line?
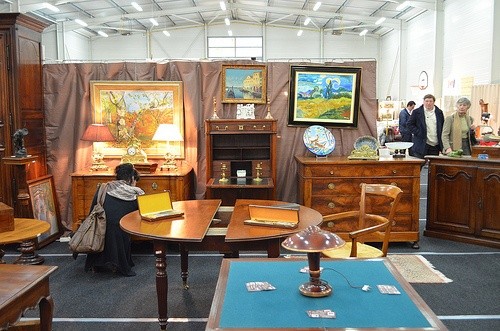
120 200 323 331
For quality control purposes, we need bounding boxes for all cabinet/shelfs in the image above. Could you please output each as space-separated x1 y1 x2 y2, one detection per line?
69 166 193 237
0 13 50 216
295 153 424 250
205 117 277 199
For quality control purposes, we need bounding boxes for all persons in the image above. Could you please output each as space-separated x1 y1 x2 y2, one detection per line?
406 94 444 172
399 101 416 156
85 163 146 277
441 98 475 155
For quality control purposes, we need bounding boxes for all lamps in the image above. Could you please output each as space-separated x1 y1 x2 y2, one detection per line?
27 0 412 37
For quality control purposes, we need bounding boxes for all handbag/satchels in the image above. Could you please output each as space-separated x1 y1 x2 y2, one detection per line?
467 129 478 146
68 204 106 254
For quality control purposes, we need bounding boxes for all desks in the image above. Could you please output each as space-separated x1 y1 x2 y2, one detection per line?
0 218 51 264
1 155 39 218
0 264 58 331
0 202 14 233
424 155 500 250
471 143 500 157
205 258 449 331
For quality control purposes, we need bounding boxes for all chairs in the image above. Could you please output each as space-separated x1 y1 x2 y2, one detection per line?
319 183 402 261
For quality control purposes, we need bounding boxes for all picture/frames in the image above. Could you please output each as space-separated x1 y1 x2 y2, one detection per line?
27 175 63 250
287 64 361 129
221 65 267 104
89 79 185 161
